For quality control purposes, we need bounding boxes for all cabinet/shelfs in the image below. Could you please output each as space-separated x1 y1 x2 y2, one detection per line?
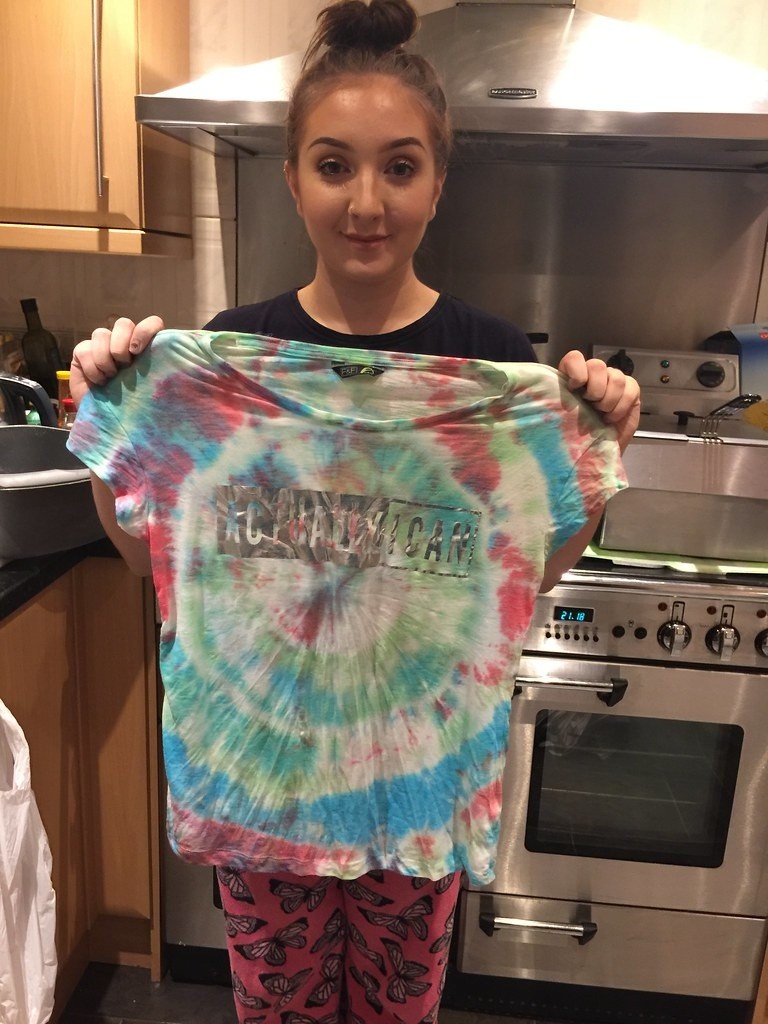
1 570 90 1024
0 0 198 263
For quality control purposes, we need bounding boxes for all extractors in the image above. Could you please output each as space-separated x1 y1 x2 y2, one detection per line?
134 0 768 178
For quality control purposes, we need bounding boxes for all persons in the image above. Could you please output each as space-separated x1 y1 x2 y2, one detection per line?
55 0 645 1024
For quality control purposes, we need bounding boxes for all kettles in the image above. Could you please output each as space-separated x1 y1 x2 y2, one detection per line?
0 371 58 430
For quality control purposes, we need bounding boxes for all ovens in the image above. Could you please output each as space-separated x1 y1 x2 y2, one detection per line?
458 560 768 1003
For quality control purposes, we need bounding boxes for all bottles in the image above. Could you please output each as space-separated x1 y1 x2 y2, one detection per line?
60 397 77 434
21 297 66 399
55 369 72 402
